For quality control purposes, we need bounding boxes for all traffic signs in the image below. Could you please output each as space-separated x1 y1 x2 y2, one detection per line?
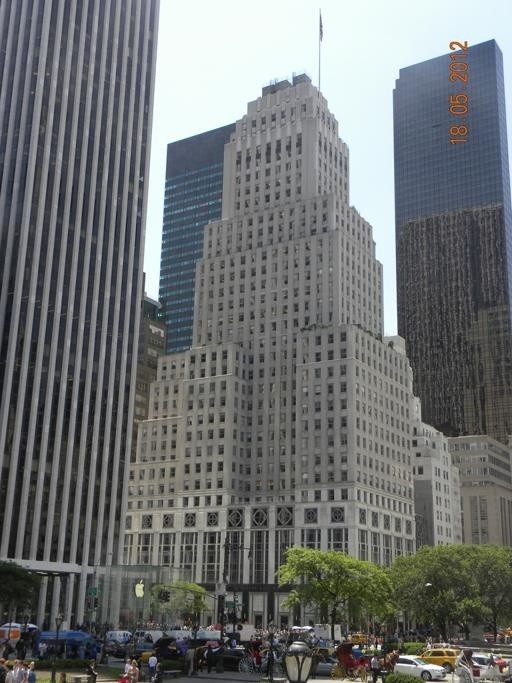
89 587 97 595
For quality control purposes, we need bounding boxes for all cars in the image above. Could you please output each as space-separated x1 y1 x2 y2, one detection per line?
311 647 334 657
390 654 448 681
134 630 148 639
470 652 506 676
416 648 463 673
209 649 249 671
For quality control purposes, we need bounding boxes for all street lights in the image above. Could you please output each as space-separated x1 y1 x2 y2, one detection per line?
100 621 109 663
267 618 278 682
216 531 256 673
279 633 324 682
90 551 114 632
23 612 30 659
50 612 65 682
190 613 202 676
424 580 448 643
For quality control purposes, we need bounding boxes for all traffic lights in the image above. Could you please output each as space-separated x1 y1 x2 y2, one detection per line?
159 589 165 600
87 597 92 608
94 597 99 608
166 591 170 602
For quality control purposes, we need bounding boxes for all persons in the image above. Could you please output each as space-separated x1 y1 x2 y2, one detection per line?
455 648 486 683
38 639 163 683
1 628 36 683
494 654 506 683
370 650 399 683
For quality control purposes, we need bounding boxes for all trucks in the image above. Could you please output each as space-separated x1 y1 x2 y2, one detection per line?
310 623 341 644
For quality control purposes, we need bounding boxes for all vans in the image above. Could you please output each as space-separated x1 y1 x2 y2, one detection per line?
106 629 133 642
350 632 370 645
